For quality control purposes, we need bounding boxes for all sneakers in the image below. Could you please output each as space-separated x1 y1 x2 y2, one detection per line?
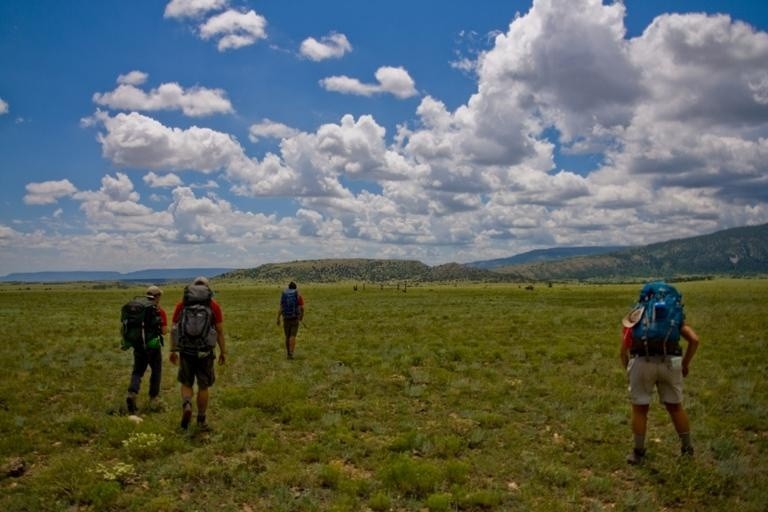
681 445 693 456
180 408 192 428
627 449 647 466
198 424 212 432
126 396 138 413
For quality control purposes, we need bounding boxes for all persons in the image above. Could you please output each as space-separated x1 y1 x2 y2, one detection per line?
127 286 167 413
169 276 225 428
277 282 304 359
621 294 699 464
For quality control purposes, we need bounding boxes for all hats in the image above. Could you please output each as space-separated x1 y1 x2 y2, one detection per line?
622 306 644 329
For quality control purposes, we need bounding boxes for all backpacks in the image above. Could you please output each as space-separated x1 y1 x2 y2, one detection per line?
632 280 683 356
121 296 158 348
281 289 300 320
176 285 217 351
